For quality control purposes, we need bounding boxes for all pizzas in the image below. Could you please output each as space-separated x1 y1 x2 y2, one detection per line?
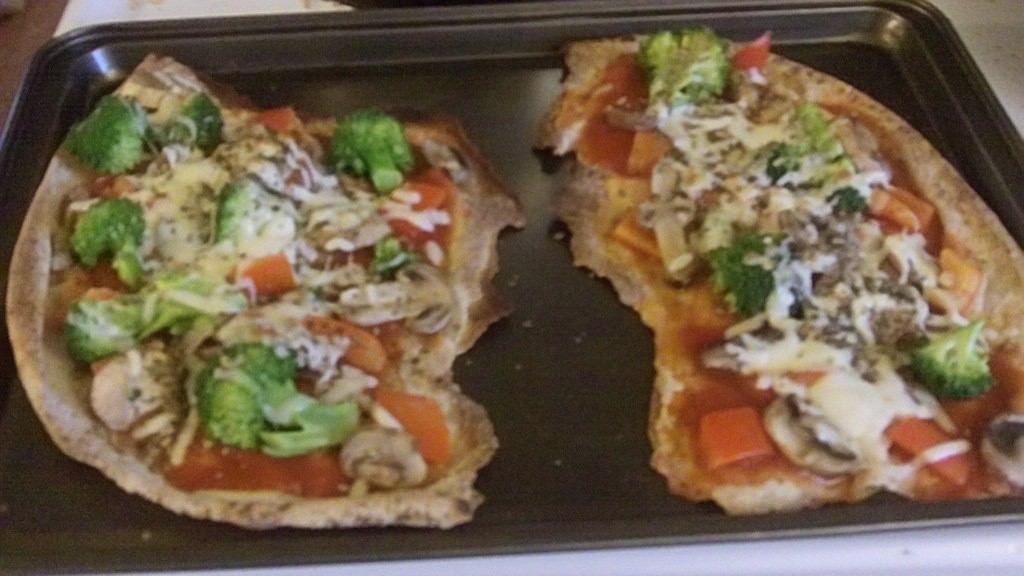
536 24 1024 516
10 51 527 529
